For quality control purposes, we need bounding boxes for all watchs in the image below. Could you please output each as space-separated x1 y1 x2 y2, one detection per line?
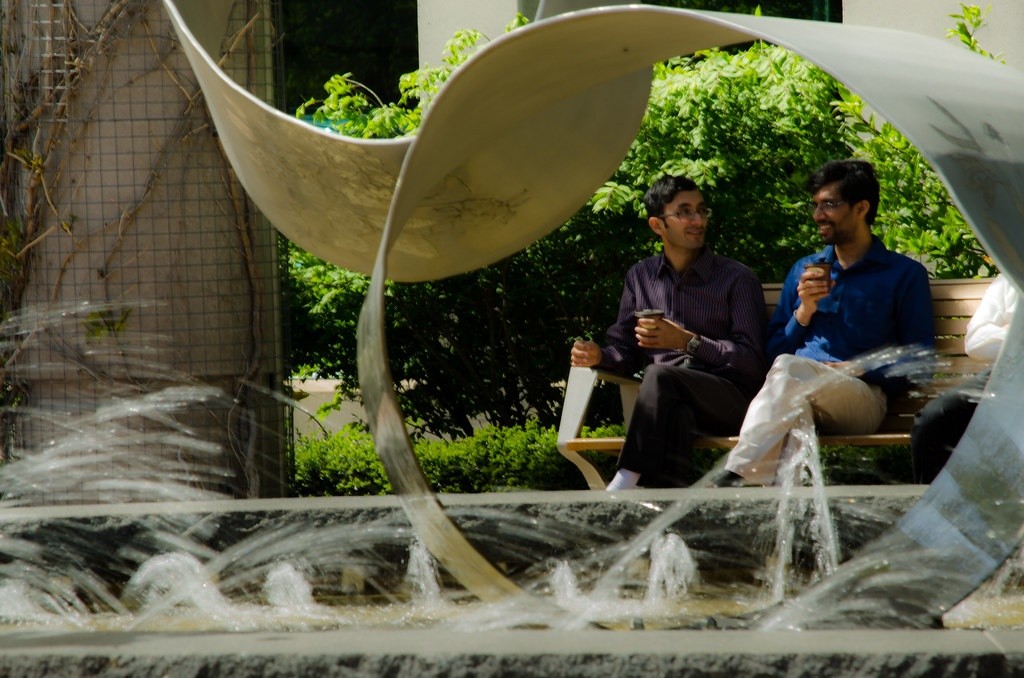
686 335 701 356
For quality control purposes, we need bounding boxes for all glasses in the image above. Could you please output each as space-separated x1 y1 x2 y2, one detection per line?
658 208 712 220
807 200 846 210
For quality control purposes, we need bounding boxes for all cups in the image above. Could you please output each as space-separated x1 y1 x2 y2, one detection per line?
634 310 665 331
805 262 832 288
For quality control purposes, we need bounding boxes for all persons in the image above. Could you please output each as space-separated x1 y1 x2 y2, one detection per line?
910 273 1018 485
688 161 934 488
570 174 766 491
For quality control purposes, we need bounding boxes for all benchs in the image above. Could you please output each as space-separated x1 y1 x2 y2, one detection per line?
556 276 997 492
